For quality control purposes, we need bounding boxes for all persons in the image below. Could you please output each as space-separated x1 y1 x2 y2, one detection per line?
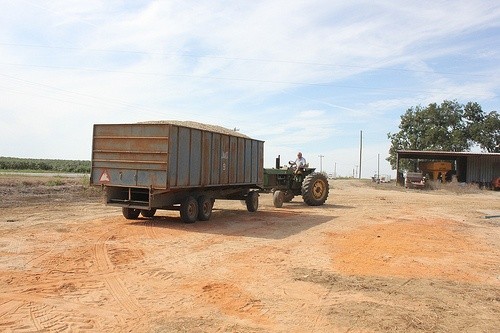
290 151 306 175
437 170 452 183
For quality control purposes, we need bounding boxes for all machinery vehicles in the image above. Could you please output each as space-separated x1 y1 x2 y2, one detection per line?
263 152 329 208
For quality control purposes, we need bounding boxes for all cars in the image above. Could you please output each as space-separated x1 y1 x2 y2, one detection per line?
405 173 425 190
